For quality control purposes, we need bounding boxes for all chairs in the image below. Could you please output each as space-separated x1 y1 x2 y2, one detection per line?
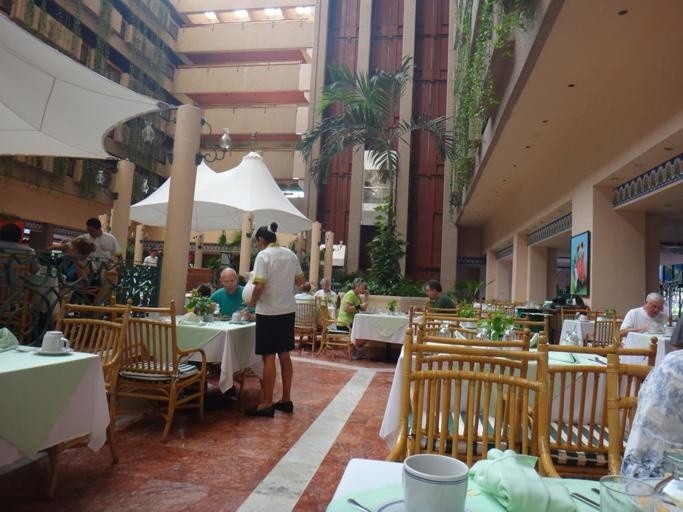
295 296 316 352
412 322 440 336
606 353 654 480
584 309 618 348
111 295 207 444
387 327 562 479
487 312 530 340
413 321 531 455
315 296 351 363
424 303 459 327
560 307 590 329
447 327 478 339
510 317 549 344
75 271 118 307
409 305 434 337
532 334 658 481
0 255 32 345
414 321 439 337
48 293 133 501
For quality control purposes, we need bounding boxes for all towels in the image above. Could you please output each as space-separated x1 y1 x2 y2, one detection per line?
469 448 578 510
0 327 18 352
549 350 577 363
178 311 200 322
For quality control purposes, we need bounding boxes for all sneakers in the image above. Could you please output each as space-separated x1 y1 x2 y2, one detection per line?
347 348 361 360
227 384 237 401
358 349 368 360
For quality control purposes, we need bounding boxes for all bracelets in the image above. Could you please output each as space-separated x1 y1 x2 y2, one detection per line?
355 304 360 310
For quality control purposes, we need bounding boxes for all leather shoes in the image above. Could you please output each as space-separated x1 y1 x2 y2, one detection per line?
272 400 293 413
245 403 275 417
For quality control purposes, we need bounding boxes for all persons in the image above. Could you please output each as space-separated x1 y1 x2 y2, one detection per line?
293 281 321 326
0 223 41 313
209 267 256 322
619 292 666 345
336 277 369 359
315 278 339 312
424 279 457 320
237 274 247 287
142 249 159 267
620 349 683 483
189 283 212 314
57 236 75 274
247 222 306 416
45 217 123 284
65 235 102 315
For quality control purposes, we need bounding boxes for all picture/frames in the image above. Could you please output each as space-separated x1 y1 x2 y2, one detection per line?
569 230 589 297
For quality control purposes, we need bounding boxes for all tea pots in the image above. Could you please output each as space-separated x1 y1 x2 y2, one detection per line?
242 271 255 302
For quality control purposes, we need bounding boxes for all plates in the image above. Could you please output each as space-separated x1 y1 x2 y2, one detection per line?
625 478 683 512
33 348 73 354
373 497 404 512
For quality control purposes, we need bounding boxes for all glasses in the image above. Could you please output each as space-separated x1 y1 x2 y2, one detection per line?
645 301 664 311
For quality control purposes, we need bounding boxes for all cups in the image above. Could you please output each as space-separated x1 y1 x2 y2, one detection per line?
664 327 676 336
231 313 241 322
402 453 469 511
502 335 512 350
41 331 69 352
599 475 655 512
543 300 553 310
647 323 660 332
